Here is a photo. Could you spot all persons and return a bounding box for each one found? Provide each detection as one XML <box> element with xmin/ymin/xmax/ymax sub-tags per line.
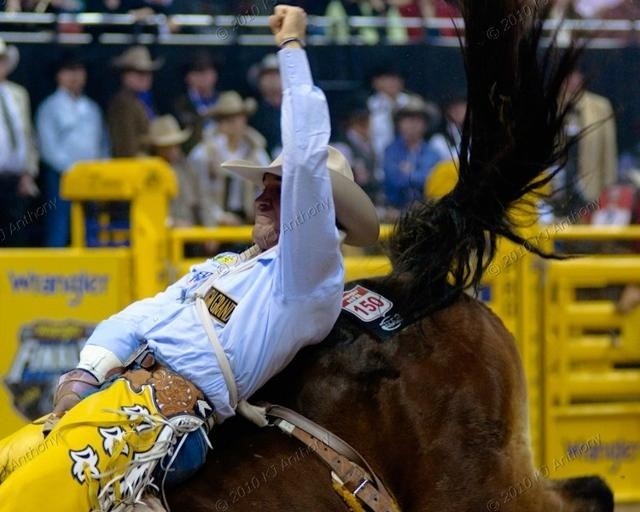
<box><xmin>0</xmin><ymin>0</ymin><xmax>639</xmax><ymax>314</ymax></box>
<box><xmin>0</xmin><ymin>4</ymin><xmax>380</xmax><ymax>512</ymax></box>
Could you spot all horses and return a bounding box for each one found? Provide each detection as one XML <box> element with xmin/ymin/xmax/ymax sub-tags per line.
<box><xmin>177</xmin><ymin>0</ymin><xmax>615</xmax><ymax>511</ymax></box>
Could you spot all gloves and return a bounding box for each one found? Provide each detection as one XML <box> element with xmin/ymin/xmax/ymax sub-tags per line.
<box><xmin>266</xmin><ymin>4</ymin><xmax>310</xmax><ymax>48</ymax></box>
<box><xmin>40</xmin><ymin>367</ymin><xmax>102</xmax><ymax>439</ymax></box>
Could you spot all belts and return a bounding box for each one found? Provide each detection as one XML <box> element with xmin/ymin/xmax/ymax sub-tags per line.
<box><xmin>121</xmin><ymin>340</ymin><xmax>159</xmax><ymax>377</ymax></box>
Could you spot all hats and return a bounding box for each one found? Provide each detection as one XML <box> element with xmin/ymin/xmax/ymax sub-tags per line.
<box><xmin>244</xmin><ymin>51</ymin><xmax>283</xmax><ymax>83</ymax></box>
<box><xmin>195</xmin><ymin>90</ymin><xmax>259</xmax><ymax>121</ymax></box>
<box><xmin>389</xmin><ymin>92</ymin><xmax>443</xmax><ymax>134</ymax></box>
<box><xmin>108</xmin><ymin>42</ymin><xmax>168</xmax><ymax>73</ymax></box>
<box><xmin>217</xmin><ymin>141</ymin><xmax>381</xmax><ymax>248</ymax></box>
<box><xmin>0</xmin><ymin>39</ymin><xmax>23</xmax><ymax>79</ymax></box>
<box><xmin>136</xmin><ymin>112</ymin><xmax>198</xmax><ymax>148</ymax></box>
